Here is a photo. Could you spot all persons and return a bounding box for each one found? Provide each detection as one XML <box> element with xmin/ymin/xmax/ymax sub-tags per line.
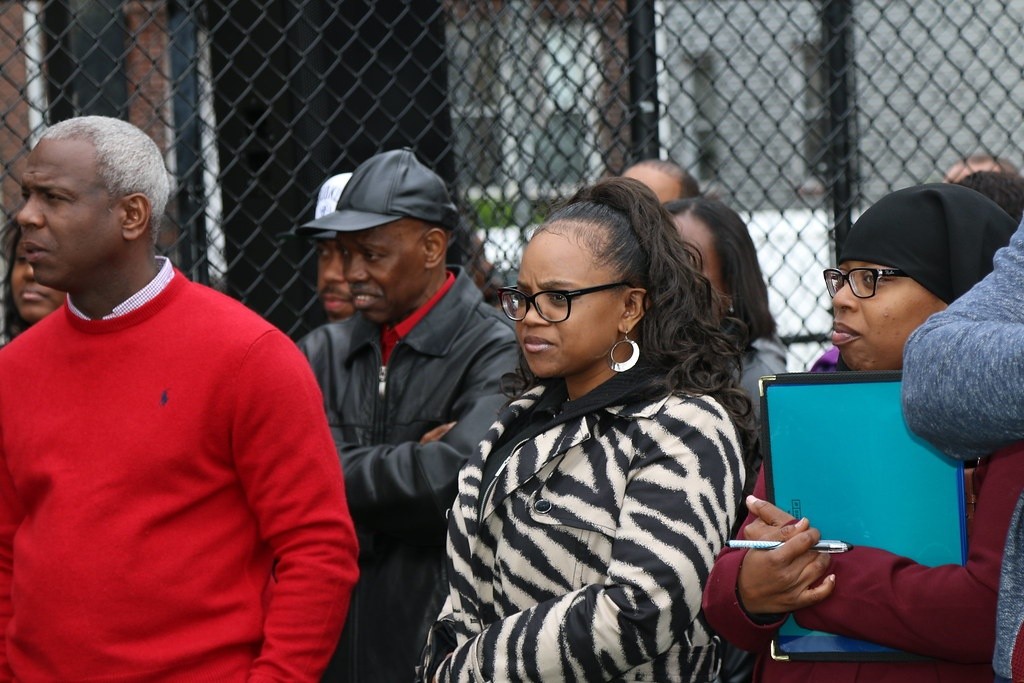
<box><xmin>619</xmin><ymin>160</ymin><xmax>787</xmax><ymax>461</ymax></box>
<box><xmin>702</xmin><ymin>182</ymin><xmax>1024</xmax><ymax>683</ymax></box>
<box><xmin>418</xmin><ymin>178</ymin><xmax>762</xmax><ymax>683</ymax></box>
<box><xmin>0</xmin><ymin>116</ymin><xmax>358</xmax><ymax>683</ymax></box>
<box><xmin>293</xmin><ymin>146</ymin><xmax>532</xmax><ymax>683</ymax></box>
<box><xmin>943</xmin><ymin>157</ymin><xmax>1024</xmax><ymax>223</ymax></box>
<box><xmin>900</xmin><ymin>206</ymin><xmax>1024</xmax><ymax>683</ymax></box>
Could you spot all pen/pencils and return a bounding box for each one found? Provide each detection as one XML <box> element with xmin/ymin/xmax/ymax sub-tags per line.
<box><xmin>723</xmin><ymin>538</ymin><xmax>853</xmax><ymax>554</ymax></box>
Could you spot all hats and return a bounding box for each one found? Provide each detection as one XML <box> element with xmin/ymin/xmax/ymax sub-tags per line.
<box><xmin>838</xmin><ymin>183</ymin><xmax>1020</xmax><ymax>307</ymax></box>
<box><xmin>277</xmin><ymin>171</ymin><xmax>354</xmax><ymax>241</ymax></box>
<box><xmin>296</xmin><ymin>146</ymin><xmax>461</xmax><ymax>231</ymax></box>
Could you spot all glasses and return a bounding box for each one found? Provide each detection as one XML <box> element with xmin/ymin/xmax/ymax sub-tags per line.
<box><xmin>497</xmin><ymin>282</ymin><xmax>648</xmax><ymax>323</ymax></box>
<box><xmin>824</xmin><ymin>268</ymin><xmax>910</xmax><ymax>299</ymax></box>
